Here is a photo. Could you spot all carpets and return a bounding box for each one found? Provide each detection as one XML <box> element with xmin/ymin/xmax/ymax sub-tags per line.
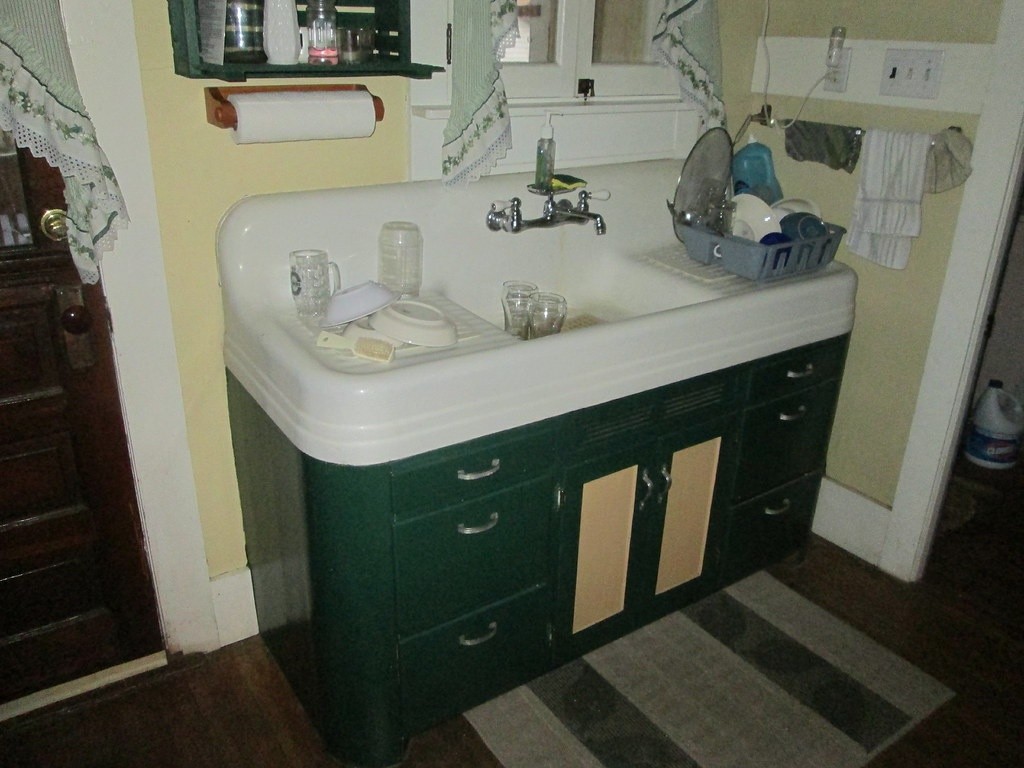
<box><xmin>461</xmin><ymin>569</ymin><xmax>962</xmax><ymax>767</ymax></box>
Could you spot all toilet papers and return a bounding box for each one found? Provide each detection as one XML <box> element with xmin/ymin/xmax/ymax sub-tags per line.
<box><xmin>227</xmin><ymin>89</ymin><xmax>378</xmax><ymax>141</ymax></box>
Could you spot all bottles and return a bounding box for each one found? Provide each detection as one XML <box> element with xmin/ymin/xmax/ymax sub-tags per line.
<box><xmin>305</xmin><ymin>0</ymin><xmax>339</xmax><ymax>56</ymax></box>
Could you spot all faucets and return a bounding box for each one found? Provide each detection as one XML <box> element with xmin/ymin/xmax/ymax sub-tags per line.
<box><xmin>542</xmin><ymin>190</ymin><xmax>606</xmax><ymax>234</ymax></box>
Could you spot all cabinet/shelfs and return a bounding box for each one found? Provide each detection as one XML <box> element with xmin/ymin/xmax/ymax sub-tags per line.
<box><xmin>218</xmin><ymin>336</ymin><xmax>853</xmax><ymax>768</ymax></box>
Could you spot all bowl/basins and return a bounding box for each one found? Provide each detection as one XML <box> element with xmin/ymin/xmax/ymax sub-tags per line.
<box><xmin>760</xmin><ymin>234</ymin><xmax>790</xmax><ymax>269</ymax></box>
<box><xmin>780</xmin><ymin>211</ymin><xmax>830</xmax><ymax>243</ymax></box>
<box><xmin>771</xmin><ymin>197</ymin><xmax>822</xmax><ymax>224</ymax></box>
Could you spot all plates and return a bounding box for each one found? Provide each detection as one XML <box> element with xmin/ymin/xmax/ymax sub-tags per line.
<box><xmin>373</xmin><ymin>294</ymin><xmax>459</xmax><ymax>348</ymax></box>
<box><xmin>730</xmin><ymin>194</ymin><xmax>782</xmax><ymax>242</ymax></box>
<box><xmin>321</xmin><ymin>280</ymin><xmax>403</xmax><ymax>328</ymax></box>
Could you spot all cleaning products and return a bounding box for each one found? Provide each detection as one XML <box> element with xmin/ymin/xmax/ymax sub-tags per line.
<box><xmin>963</xmin><ymin>379</ymin><xmax>1024</xmax><ymax>469</ymax></box>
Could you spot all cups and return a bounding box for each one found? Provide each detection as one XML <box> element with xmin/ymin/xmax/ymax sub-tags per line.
<box><xmin>336</xmin><ymin>27</ymin><xmax>376</xmax><ymax>65</ymax></box>
<box><xmin>502</xmin><ymin>277</ymin><xmax>539</xmax><ymax>341</ymax></box>
<box><xmin>529</xmin><ymin>292</ymin><xmax>568</xmax><ymax>338</ymax></box>
<box><xmin>286</xmin><ymin>248</ymin><xmax>341</xmax><ymax>318</ymax></box>
<box><xmin>380</xmin><ymin>221</ymin><xmax>424</xmax><ymax>300</ymax></box>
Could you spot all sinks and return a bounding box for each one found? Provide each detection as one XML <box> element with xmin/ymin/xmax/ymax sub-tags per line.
<box><xmin>427</xmin><ymin>237</ymin><xmax>716</xmax><ymax>348</ymax></box>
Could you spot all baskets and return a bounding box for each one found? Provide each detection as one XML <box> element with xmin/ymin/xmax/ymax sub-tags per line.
<box><xmin>677</xmin><ymin>219</ymin><xmax>847</xmax><ymax>282</ymax></box>
